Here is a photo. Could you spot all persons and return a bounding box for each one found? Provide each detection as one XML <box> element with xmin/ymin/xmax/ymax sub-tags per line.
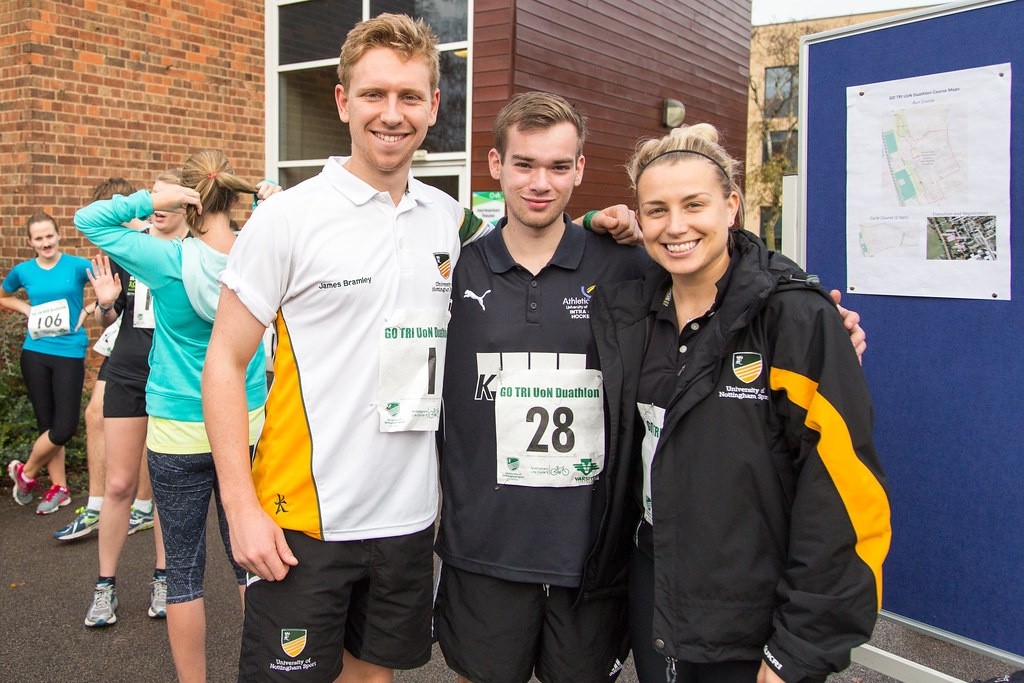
<box><xmin>201</xmin><ymin>12</ymin><xmax>644</xmax><ymax>683</ymax></box>
<box><xmin>570</xmin><ymin>123</ymin><xmax>893</xmax><ymax>683</ymax></box>
<box><xmin>74</xmin><ymin>151</ymin><xmax>282</xmax><ymax>682</ymax></box>
<box><xmin>435</xmin><ymin>91</ymin><xmax>866</xmax><ymax>683</ymax></box>
<box><xmin>0</xmin><ymin>169</ymin><xmax>195</xmax><ymax>626</ymax></box>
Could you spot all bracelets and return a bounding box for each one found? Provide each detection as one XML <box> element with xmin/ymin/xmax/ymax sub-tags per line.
<box><xmin>583</xmin><ymin>210</ymin><xmax>599</xmax><ymax>232</ymax></box>
<box><xmin>99</xmin><ymin>305</ymin><xmax>112</xmax><ymax>314</ymax></box>
<box><xmin>84</xmin><ymin>307</ymin><xmax>90</xmax><ymax>315</ymax></box>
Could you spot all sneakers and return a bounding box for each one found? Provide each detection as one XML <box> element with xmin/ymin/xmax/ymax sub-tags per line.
<box><xmin>53</xmin><ymin>507</ymin><xmax>100</xmax><ymax>541</ymax></box>
<box><xmin>36</xmin><ymin>484</ymin><xmax>72</xmax><ymax>515</ymax></box>
<box><xmin>84</xmin><ymin>578</ymin><xmax>118</xmax><ymax>627</ymax></box>
<box><xmin>127</xmin><ymin>504</ymin><xmax>154</xmax><ymax>535</ymax></box>
<box><xmin>148</xmin><ymin>570</ymin><xmax>166</xmax><ymax>618</ymax></box>
<box><xmin>8</xmin><ymin>460</ymin><xmax>38</xmax><ymax>506</ymax></box>
